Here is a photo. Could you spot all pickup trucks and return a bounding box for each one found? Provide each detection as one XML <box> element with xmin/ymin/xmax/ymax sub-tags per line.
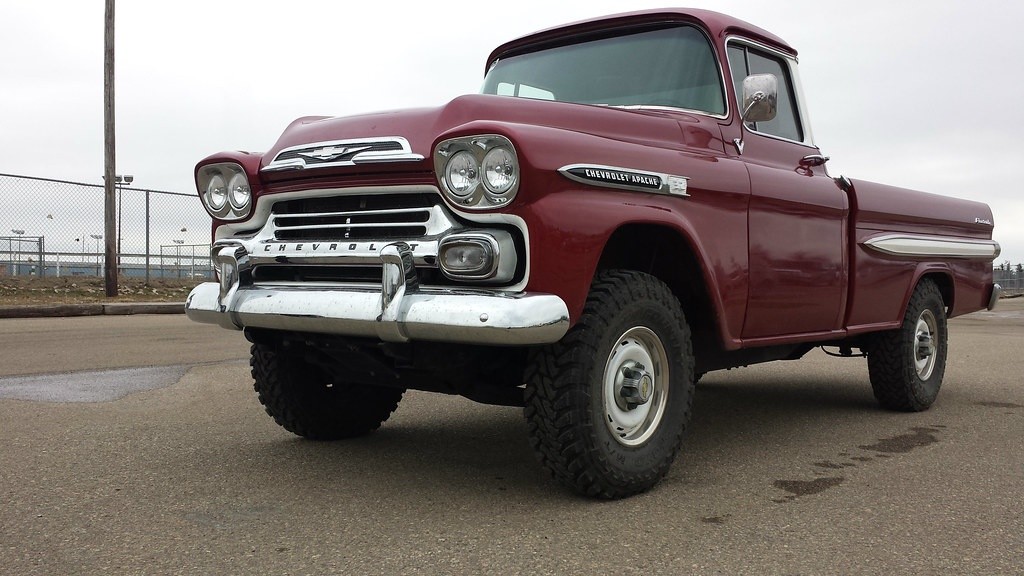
<box><xmin>183</xmin><ymin>8</ymin><xmax>1002</xmax><ymax>503</ymax></box>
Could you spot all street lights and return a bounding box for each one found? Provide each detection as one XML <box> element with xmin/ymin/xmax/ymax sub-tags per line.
<box><xmin>11</xmin><ymin>229</ymin><xmax>25</xmax><ymax>275</ymax></box>
<box><xmin>91</xmin><ymin>234</ymin><xmax>103</xmax><ymax>277</ymax></box>
<box><xmin>173</xmin><ymin>239</ymin><xmax>184</xmax><ymax>281</ymax></box>
<box><xmin>46</xmin><ymin>214</ymin><xmax>53</xmax><ymax>260</ymax></box>
<box><xmin>180</xmin><ymin>228</ymin><xmax>188</xmax><ymax>279</ymax></box>
<box><xmin>103</xmin><ymin>175</ymin><xmax>133</xmax><ymax>275</ymax></box>
